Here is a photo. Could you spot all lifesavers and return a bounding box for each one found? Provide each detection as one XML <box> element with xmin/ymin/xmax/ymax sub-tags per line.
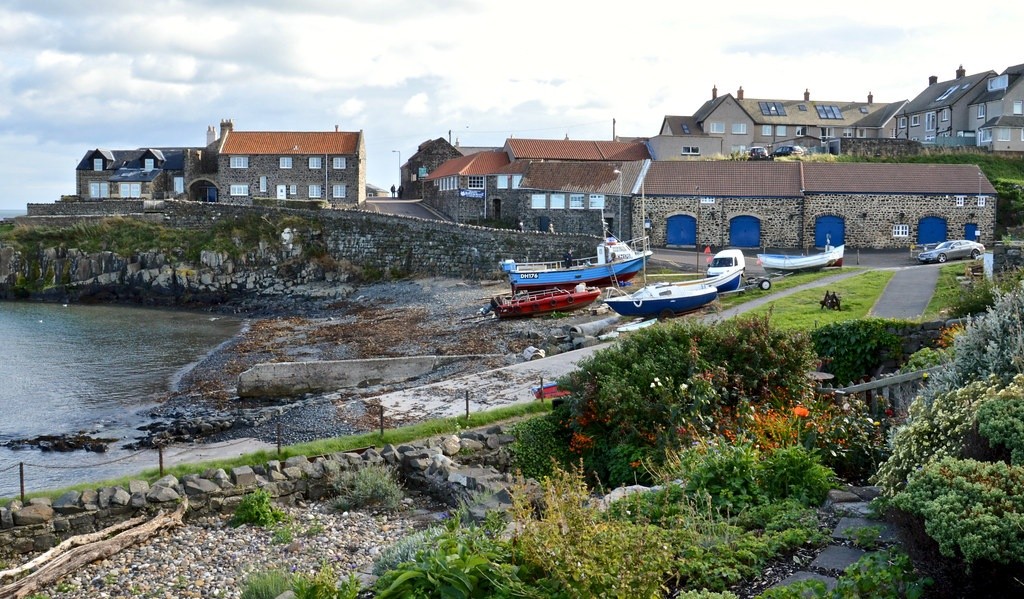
<box><xmin>515</xmin><ymin>306</ymin><xmax>522</xmax><ymax>313</ymax></box>
<box><xmin>532</xmin><ymin>302</ymin><xmax>540</xmax><ymax>310</ymax></box>
<box><xmin>549</xmin><ymin>299</ymin><xmax>557</xmax><ymax>307</ymax></box>
<box><xmin>481</xmin><ymin>303</ymin><xmax>493</xmax><ymax>315</ymax></box>
<box><xmin>566</xmin><ymin>296</ymin><xmax>575</xmax><ymax>303</ymax></box>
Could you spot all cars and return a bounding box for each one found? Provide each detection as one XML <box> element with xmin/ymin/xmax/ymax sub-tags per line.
<box><xmin>768</xmin><ymin>145</ymin><xmax>805</xmax><ymax>160</ymax></box>
<box><xmin>918</xmin><ymin>240</ymin><xmax>986</xmax><ymax>262</ymax></box>
<box><xmin>747</xmin><ymin>146</ymin><xmax>769</xmax><ymax>160</ymax></box>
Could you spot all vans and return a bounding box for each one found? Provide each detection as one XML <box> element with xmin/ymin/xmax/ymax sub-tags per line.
<box><xmin>707</xmin><ymin>249</ymin><xmax>746</xmax><ymax>279</ymax></box>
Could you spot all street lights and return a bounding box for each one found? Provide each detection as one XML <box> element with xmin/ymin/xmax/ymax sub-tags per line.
<box><xmin>613</xmin><ymin>170</ymin><xmax>622</xmax><ymax>243</ymax></box>
<box><xmin>392</xmin><ymin>150</ymin><xmax>400</xmax><ymax>187</ymax></box>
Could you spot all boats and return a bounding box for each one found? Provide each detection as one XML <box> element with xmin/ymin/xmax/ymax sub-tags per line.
<box><xmin>499</xmin><ymin>237</ymin><xmax>653</xmax><ymax>288</ymax></box>
<box><xmin>756</xmin><ymin>243</ymin><xmax>844</xmax><ymax>275</ymax></box>
<box><xmin>650</xmin><ymin>266</ymin><xmax>744</xmax><ymax>294</ymax></box>
<box><xmin>603</xmin><ymin>281</ymin><xmax>718</xmax><ymax>318</ymax></box>
<box><xmin>490</xmin><ymin>282</ymin><xmax>602</xmax><ymax>319</ymax></box>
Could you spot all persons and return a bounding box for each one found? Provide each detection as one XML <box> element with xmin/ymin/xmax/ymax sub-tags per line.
<box><xmin>390</xmin><ymin>184</ymin><xmax>396</xmax><ymax>198</ymax></box>
<box><xmin>397</xmin><ymin>185</ymin><xmax>404</xmax><ymax>199</ymax></box>
<box><xmin>564</xmin><ymin>248</ymin><xmax>573</xmax><ymax>269</ymax></box>
